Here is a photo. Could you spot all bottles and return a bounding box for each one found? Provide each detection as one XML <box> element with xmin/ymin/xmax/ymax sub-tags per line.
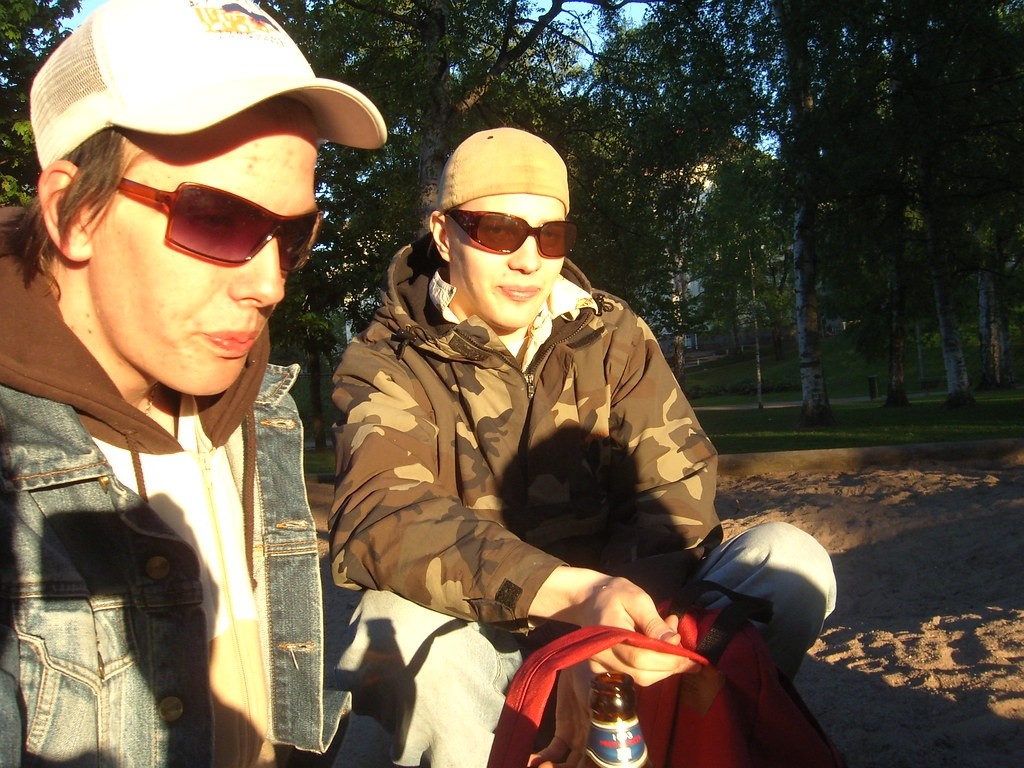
<box><xmin>576</xmin><ymin>670</ymin><xmax>653</xmax><ymax>768</ymax></box>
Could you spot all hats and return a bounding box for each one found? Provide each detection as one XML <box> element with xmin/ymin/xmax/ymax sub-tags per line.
<box><xmin>437</xmin><ymin>128</ymin><xmax>570</xmax><ymax>217</ymax></box>
<box><xmin>30</xmin><ymin>0</ymin><xmax>388</xmax><ymax>171</ymax></box>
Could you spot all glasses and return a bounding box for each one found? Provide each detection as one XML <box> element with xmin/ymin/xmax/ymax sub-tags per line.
<box><xmin>444</xmin><ymin>209</ymin><xmax>578</xmax><ymax>257</ymax></box>
<box><xmin>77</xmin><ymin>164</ymin><xmax>325</xmax><ymax>272</ymax></box>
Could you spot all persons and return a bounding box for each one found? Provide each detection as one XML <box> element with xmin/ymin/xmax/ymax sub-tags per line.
<box><xmin>0</xmin><ymin>0</ymin><xmax>388</xmax><ymax>768</ymax></box>
<box><xmin>328</xmin><ymin>126</ymin><xmax>837</xmax><ymax>767</ymax></box>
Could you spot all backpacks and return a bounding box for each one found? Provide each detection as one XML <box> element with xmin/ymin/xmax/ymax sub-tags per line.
<box><xmin>487</xmin><ymin>580</ymin><xmax>846</xmax><ymax>768</ymax></box>
